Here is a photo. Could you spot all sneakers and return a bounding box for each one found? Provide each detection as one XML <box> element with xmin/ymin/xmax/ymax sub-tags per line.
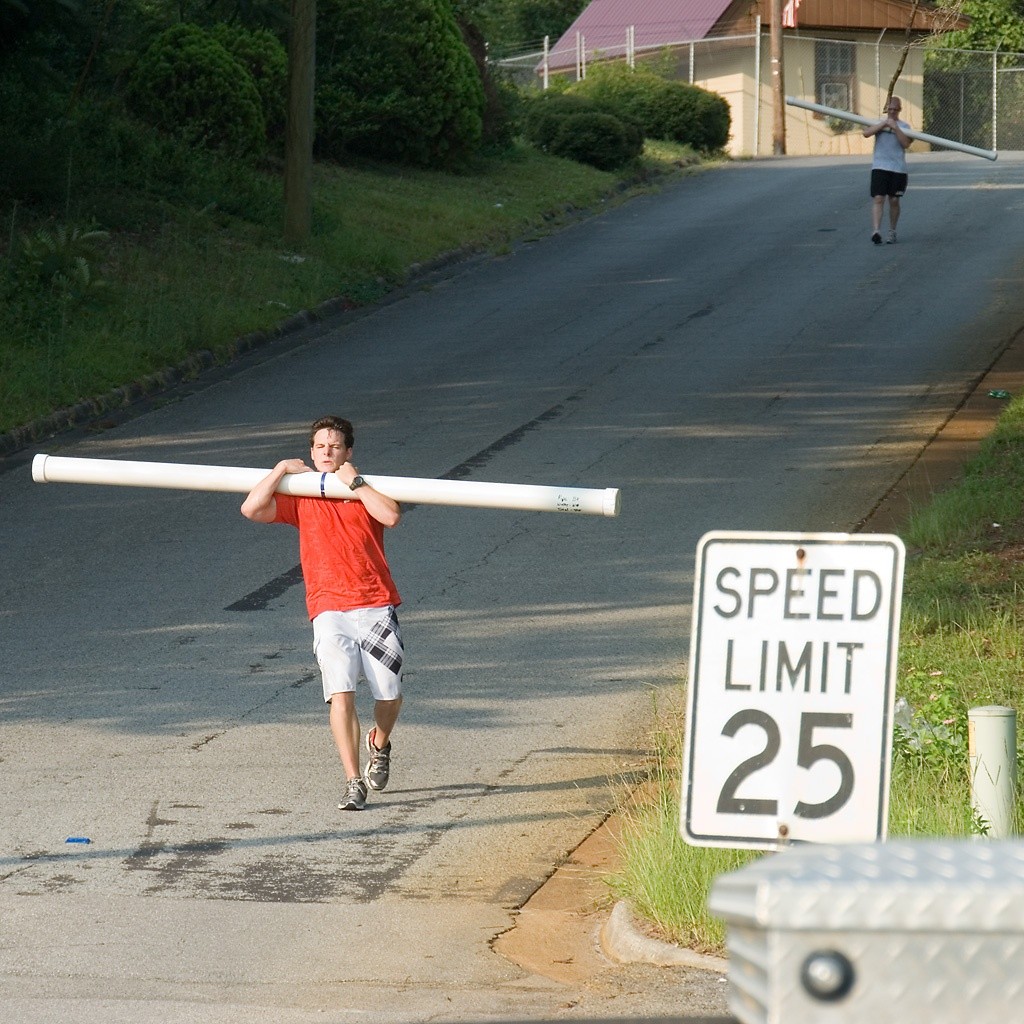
<box><xmin>872</xmin><ymin>232</ymin><xmax>883</xmax><ymax>244</ymax></box>
<box><xmin>886</xmin><ymin>229</ymin><xmax>895</xmax><ymax>243</ymax></box>
<box><xmin>364</xmin><ymin>725</ymin><xmax>391</xmax><ymax>791</ymax></box>
<box><xmin>337</xmin><ymin>778</ymin><xmax>369</xmax><ymax>811</ymax></box>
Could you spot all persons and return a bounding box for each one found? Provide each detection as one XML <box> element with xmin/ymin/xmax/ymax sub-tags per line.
<box><xmin>241</xmin><ymin>414</ymin><xmax>405</xmax><ymax>812</ymax></box>
<box><xmin>862</xmin><ymin>96</ymin><xmax>914</xmax><ymax>244</ymax></box>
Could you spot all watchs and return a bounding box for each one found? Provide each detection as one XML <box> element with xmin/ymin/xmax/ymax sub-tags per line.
<box><xmin>349</xmin><ymin>475</ymin><xmax>363</xmax><ymax>491</ymax></box>
